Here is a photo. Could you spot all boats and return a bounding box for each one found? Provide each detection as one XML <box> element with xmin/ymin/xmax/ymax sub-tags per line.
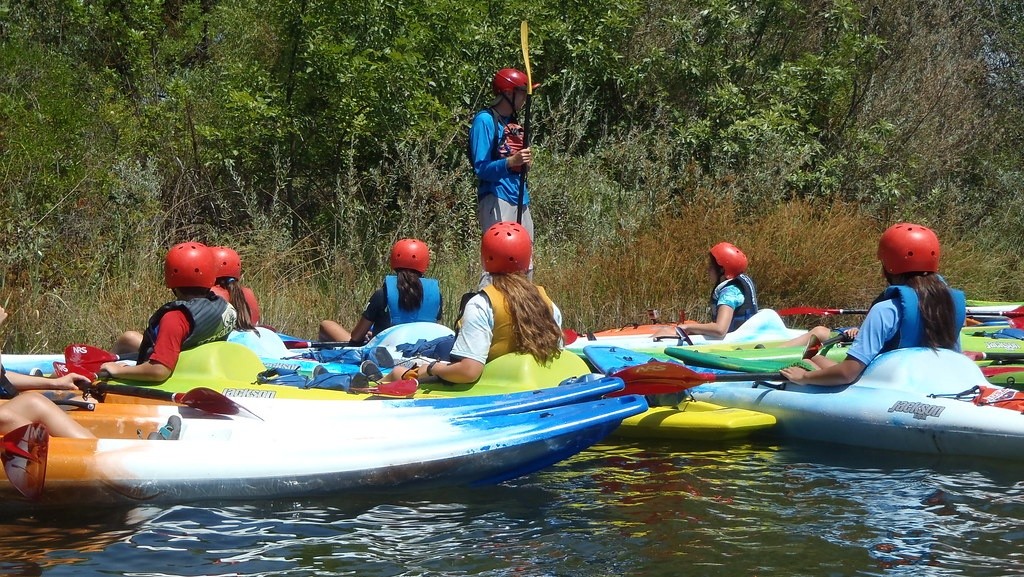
<box><xmin>0</xmin><ymin>299</ymin><xmax>1024</xmax><ymax>515</ymax></box>
<box><xmin>583</xmin><ymin>343</ymin><xmax>1024</xmax><ymax>459</ymax></box>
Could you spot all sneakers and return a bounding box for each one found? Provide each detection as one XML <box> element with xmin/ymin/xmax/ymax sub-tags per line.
<box><xmin>360</xmin><ymin>360</ymin><xmax>383</xmax><ymax>383</ymax></box>
<box><xmin>138</xmin><ymin>415</ymin><xmax>181</xmax><ymax>440</ymax></box>
<box><xmin>312</xmin><ymin>365</ymin><xmax>329</xmax><ymax>380</ymax></box>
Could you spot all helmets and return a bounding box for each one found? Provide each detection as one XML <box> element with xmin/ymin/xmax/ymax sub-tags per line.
<box><xmin>494</xmin><ymin>68</ymin><xmax>541</xmax><ymax>96</ymax></box>
<box><xmin>877</xmin><ymin>223</ymin><xmax>939</xmax><ymax>275</ymax></box>
<box><xmin>710</xmin><ymin>242</ymin><xmax>748</xmax><ymax>279</ymax></box>
<box><xmin>392</xmin><ymin>238</ymin><xmax>430</xmax><ymax>273</ymax></box>
<box><xmin>481</xmin><ymin>221</ymin><xmax>533</xmax><ymax>273</ymax></box>
<box><xmin>210</xmin><ymin>245</ymin><xmax>241</xmax><ymax>281</ymax></box>
<box><xmin>165</xmin><ymin>242</ymin><xmax>216</xmax><ymax>289</ymax></box>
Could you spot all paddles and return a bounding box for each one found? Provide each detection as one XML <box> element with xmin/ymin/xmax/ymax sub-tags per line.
<box><xmin>515</xmin><ymin>19</ymin><xmax>534</xmax><ymax>224</ymax></box>
<box><xmin>283</xmin><ymin>339</ymin><xmax>369</xmax><ymax>349</ymax></box>
<box><xmin>612</xmin><ymin>361</ymin><xmax>1023</xmax><ymax>394</ymax></box>
<box><xmin>801</xmin><ymin>333</ymin><xmax>847</xmax><ymax>360</ymax></box>
<box><xmin>74</xmin><ymin>379</ymin><xmax>266</xmax><ymax>423</ymax></box>
<box><xmin>777</xmin><ymin>306</ymin><xmax>1024</xmax><ymax>329</ymax></box>
<box><xmin>53</xmin><ymin>344</ymin><xmax>140</xmax><ymax>382</ymax></box>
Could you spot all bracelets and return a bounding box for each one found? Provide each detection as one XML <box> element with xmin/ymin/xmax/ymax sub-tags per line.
<box><xmin>428</xmin><ymin>361</ymin><xmax>439</xmax><ymax>376</ymax></box>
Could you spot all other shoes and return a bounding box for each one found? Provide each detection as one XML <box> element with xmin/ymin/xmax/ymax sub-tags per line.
<box><xmin>29</xmin><ymin>367</ymin><xmax>43</xmax><ymax>377</ymax></box>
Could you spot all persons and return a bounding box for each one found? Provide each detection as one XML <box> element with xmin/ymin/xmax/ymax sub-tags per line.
<box><xmin>414</xmin><ymin>222</ymin><xmax>563</xmax><ymax>384</ymax></box>
<box><xmin>782</xmin><ymin>221</ymin><xmax>967</xmax><ymax>388</ymax></box>
<box><xmin>206</xmin><ymin>242</ymin><xmax>261</xmax><ymax>329</ymax></box>
<box><xmin>101</xmin><ymin>242</ymin><xmax>237</xmax><ymax>381</ymax></box>
<box><xmin>470</xmin><ymin>68</ymin><xmax>534</xmax><ymax>284</ymax></box>
<box><xmin>679</xmin><ymin>240</ymin><xmax>757</xmax><ymax>339</ymax></box>
<box><xmin>0</xmin><ymin>307</ymin><xmax>183</xmax><ymax>439</ymax></box>
<box><xmin>317</xmin><ymin>237</ymin><xmax>441</xmax><ymax>347</ymax></box>
